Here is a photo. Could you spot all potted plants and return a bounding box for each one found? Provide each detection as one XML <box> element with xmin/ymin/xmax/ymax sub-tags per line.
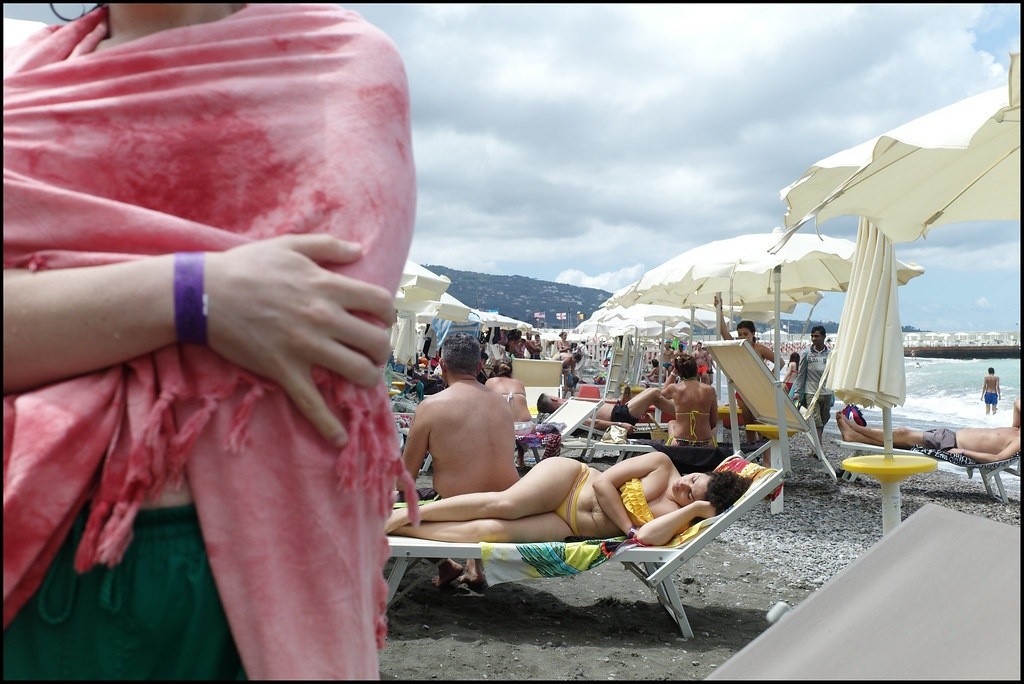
<box><xmin>593</xmin><ymin>372</ymin><xmax>608</xmax><ymax>385</ymax></box>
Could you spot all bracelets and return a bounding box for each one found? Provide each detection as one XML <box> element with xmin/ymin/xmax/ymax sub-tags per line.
<box><xmin>174</xmin><ymin>251</ymin><xmax>207</xmax><ymax>345</ymax></box>
<box><xmin>627</xmin><ymin>525</ymin><xmax>637</xmax><ymax>539</ymax></box>
<box><xmin>960</xmin><ymin>449</ymin><xmax>966</xmax><ymax>455</ymax></box>
<box><xmin>619</xmin><ymin>422</ymin><xmax>621</xmax><ymax>426</ymax></box>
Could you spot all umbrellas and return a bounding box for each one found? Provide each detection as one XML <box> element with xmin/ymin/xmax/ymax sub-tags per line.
<box><xmin>393</xmin><ymin>259</ymin><xmax>533</xmax><ymax>378</ymax></box>
<box><xmin>629</xmin><ymin>225</ymin><xmax>925</xmax><ymax>381</ymax></box>
<box><xmin>801</xmin><ymin>221</ymin><xmax>906</xmax><ymax>459</ymax></box>
<box><xmin>574</xmin><ymin>280</ymin><xmax>736</xmax><ymax>423</ymax></box>
<box><xmin>767</xmin><ymin>52</ymin><xmax>1020</xmax><ymax>254</ymax></box>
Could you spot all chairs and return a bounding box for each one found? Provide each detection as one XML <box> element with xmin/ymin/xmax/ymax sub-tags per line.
<box><xmin>387</xmin><ymin>358</ymin><xmax>1020</xmax><ymax>680</ymax></box>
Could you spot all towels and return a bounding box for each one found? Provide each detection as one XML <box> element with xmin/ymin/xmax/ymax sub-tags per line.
<box><xmin>649</xmin><ymin>438</ymin><xmax>769</xmax><ymax>473</ymax></box>
<box><xmin>913</xmin><ymin>444</ymin><xmax>1020</xmax><ymax>478</ymax></box>
<box><xmin>479</xmin><ymin>459</ymin><xmax>783</xmax><ymax>587</ymax></box>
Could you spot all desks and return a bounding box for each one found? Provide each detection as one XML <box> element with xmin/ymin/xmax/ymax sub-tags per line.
<box><xmin>718</xmin><ymin>407</ymin><xmax>744</xmax><ymax>443</ymax></box>
<box><xmin>842</xmin><ymin>455</ymin><xmax>938</xmax><ymax>537</ymax></box>
<box><xmin>745</xmin><ymin>424</ymin><xmax>800</xmax><ymax>514</ymax></box>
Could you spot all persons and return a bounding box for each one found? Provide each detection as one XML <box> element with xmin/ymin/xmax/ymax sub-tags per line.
<box><xmin>383</xmin><ymin>451</ymin><xmax>753</xmax><ymax>546</ymax></box>
<box><xmin>714</xmin><ymin>295</ymin><xmax>785</xmax><ymax>445</ymax></box>
<box><xmin>836</xmin><ymin>397</ymin><xmax>1020</xmax><ymax>464</ymax></box>
<box><xmin>401</xmin><ymin>334</ymin><xmax>520</xmax><ymax>498</ymax></box>
<box><xmin>981</xmin><ymin>368</ymin><xmax>1001</xmax><ymax>415</ymax></box>
<box><xmin>4</xmin><ymin>4</ymin><xmax>417</xmax><ymax>680</ymax></box>
<box><xmin>403</xmin><ymin>325</ymin><xmax>833</xmax><ymax>459</ymax></box>
